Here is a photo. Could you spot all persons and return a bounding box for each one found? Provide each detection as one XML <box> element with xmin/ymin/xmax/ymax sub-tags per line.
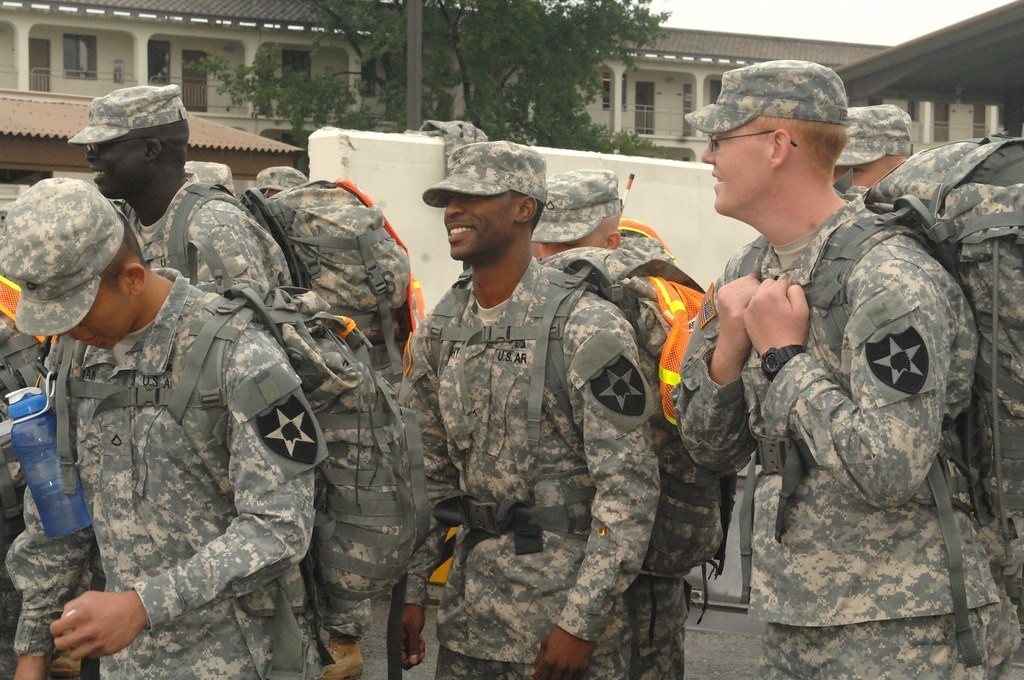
<box><xmin>833</xmin><ymin>104</ymin><xmax>913</xmax><ymax>189</ymax></box>
<box><xmin>256</xmin><ymin>166</ymin><xmax>372</xmax><ymax>680</ymax></box>
<box><xmin>183</xmin><ymin>162</ymin><xmax>235</xmax><ymax>197</ymax></box>
<box><xmin>0</xmin><ymin>177</ymin><xmax>330</xmax><ymax>680</ymax></box>
<box><xmin>66</xmin><ymin>84</ymin><xmax>293</xmax><ymax>304</ymax></box>
<box><xmin>529</xmin><ymin>170</ymin><xmax>690</xmax><ymax>680</ymax></box>
<box><xmin>399</xmin><ymin>139</ymin><xmax>661</xmax><ymax>680</ymax></box>
<box><xmin>667</xmin><ymin>59</ymin><xmax>1022</xmax><ymax>680</ymax></box>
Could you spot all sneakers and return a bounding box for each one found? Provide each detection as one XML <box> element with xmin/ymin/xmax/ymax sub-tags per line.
<box><xmin>321</xmin><ymin>633</ymin><xmax>364</xmax><ymax>680</ymax></box>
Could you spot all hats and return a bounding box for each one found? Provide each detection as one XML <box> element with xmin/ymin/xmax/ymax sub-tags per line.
<box><xmin>0</xmin><ymin>178</ymin><xmax>124</xmax><ymax>337</ymax></box>
<box><xmin>422</xmin><ymin>141</ymin><xmax>548</xmax><ymax>207</ymax></box>
<box><xmin>836</xmin><ymin>105</ymin><xmax>913</xmax><ymax>166</ymax></box>
<box><xmin>256</xmin><ymin>166</ymin><xmax>308</xmax><ymax>190</ymax></box>
<box><xmin>68</xmin><ymin>83</ymin><xmax>188</xmax><ymax>146</ymax></box>
<box><xmin>685</xmin><ymin>60</ymin><xmax>848</xmax><ymax>136</ymax></box>
<box><xmin>532</xmin><ymin>170</ymin><xmax>621</xmax><ymax>243</ymax></box>
<box><xmin>185</xmin><ymin>161</ymin><xmax>236</xmax><ymax>197</ymax></box>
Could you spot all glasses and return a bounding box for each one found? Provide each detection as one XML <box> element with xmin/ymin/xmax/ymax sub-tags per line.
<box><xmin>87</xmin><ymin>135</ymin><xmax>166</xmax><ymax>155</ymax></box>
<box><xmin>709</xmin><ymin>130</ymin><xmax>797</xmax><ymax>152</ymax></box>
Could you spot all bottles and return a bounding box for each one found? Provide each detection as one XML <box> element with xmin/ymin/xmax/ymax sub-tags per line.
<box><xmin>4</xmin><ymin>371</ymin><xmax>91</xmax><ymax>537</ymax></box>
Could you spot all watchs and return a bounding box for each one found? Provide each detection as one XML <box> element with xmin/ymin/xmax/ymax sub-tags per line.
<box><xmin>761</xmin><ymin>343</ymin><xmax>805</xmax><ymax>382</ymax></box>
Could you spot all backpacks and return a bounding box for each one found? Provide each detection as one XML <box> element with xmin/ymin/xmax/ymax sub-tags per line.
<box><xmin>58</xmin><ymin>280</ymin><xmax>418</xmax><ymax>598</ymax></box>
<box><xmin>425</xmin><ymin>247</ymin><xmax>723</xmax><ymax>578</ymax></box>
<box><xmin>739</xmin><ymin>138</ymin><xmax>1024</xmax><ymax>665</ymax></box>
<box><xmin>172</xmin><ymin>181</ymin><xmax>412</xmax><ymax>375</ymax></box>
